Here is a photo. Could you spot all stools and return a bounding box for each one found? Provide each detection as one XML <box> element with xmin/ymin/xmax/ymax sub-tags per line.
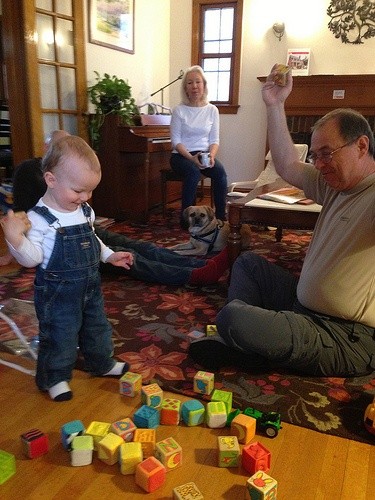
<box><xmin>159</xmin><ymin>169</ymin><xmax>214</xmax><ymax>218</ymax></box>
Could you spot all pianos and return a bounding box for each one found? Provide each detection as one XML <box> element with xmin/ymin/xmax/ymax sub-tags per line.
<box><xmin>89</xmin><ymin>113</ymin><xmax>181</xmax><ymax>224</ymax></box>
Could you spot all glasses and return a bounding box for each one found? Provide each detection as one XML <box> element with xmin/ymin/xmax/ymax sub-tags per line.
<box><xmin>306</xmin><ymin>137</ymin><xmax>361</xmax><ymax>163</ymax></box>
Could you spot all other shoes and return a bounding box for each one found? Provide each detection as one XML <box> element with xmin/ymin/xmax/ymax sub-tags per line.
<box><xmin>180</xmin><ymin>214</ymin><xmax>187</xmax><ymax>227</ymax></box>
<box><xmin>188</xmin><ymin>335</ymin><xmax>273</xmax><ymax>375</ymax></box>
<box><xmin>216</xmin><ymin>217</ymin><xmax>227</xmax><ymax>221</ymax></box>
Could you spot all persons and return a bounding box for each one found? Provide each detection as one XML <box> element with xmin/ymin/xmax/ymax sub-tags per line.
<box><xmin>13</xmin><ymin>129</ymin><xmax>231</xmax><ymax>289</ymax></box>
<box><xmin>168</xmin><ymin>65</ymin><xmax>228</xmax><ymax>221</ymax></box>
<box><xmin>187</xmin><ymin>63</ymin><xmax>375</xmax><ymax>383</ymax></box>
<box><xmin>1</xmin><ymin>136</ymin><xmax>134</xmax><ymax>403</ymax></box>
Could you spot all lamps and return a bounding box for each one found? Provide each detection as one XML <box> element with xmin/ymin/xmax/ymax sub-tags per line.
<box><xmin>271</xmin><ymin>22</ymin><xmax>285</xmax><ymax>41</ymax></box>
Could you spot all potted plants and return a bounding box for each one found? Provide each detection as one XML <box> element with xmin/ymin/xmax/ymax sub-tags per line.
<box><xmin>85</xmin><ymin>70</ymin><xmax>140</xmax><ymax>152</ymax></box>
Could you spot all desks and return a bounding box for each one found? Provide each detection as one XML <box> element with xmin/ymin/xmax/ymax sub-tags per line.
<box><xmin>227</xmin><ymin>184</ymin><xmax>324</xmax><ymax>293</ymax></box>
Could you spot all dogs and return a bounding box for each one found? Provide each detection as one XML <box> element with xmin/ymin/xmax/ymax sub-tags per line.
<box><xmin>165</xmin><ymin>203</ymin><xmax>253</xmax><ymax>255</ymax></box>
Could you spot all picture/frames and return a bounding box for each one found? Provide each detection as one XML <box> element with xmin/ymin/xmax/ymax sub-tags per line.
<box><xmin>88</xmin><ymin>0</ymin><xmax>136</xmax><ymax>55</ymax></box>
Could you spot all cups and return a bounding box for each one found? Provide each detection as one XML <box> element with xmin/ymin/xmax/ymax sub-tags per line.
<box><xmin>200</xmin><ymin>153</ymin><xmax>210</xmax><ymax>167</ymax></box>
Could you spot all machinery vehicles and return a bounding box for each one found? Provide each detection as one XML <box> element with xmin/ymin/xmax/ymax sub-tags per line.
<box><xmin>226</xmin><ymin>406</ymin><xmax>283</xmax><ymax>439</ymax></box>
